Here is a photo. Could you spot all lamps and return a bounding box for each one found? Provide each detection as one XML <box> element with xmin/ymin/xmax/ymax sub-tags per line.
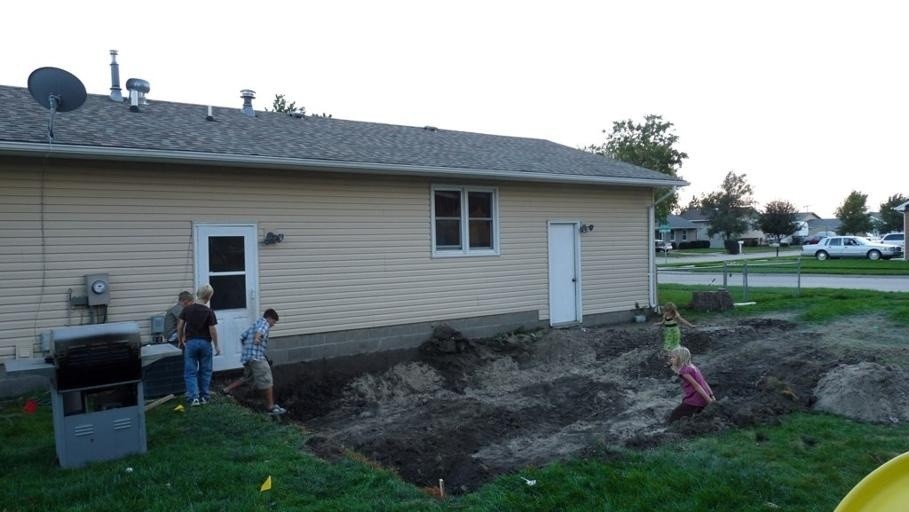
<box><xmin>264</xmin><ymin>232</ymin><xmax>284</xmax><ymax>246</ymax></box>
<box><xmin>580</xmin><ymin>224</ymin><xmax>594</xmax><ymax>234</ymax></box>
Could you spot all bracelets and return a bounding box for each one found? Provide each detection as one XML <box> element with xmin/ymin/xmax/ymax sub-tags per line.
<box><xmin>710</xmin><ymin>393</ymin><xmax>715</xmax><ymax>397</ymax></box>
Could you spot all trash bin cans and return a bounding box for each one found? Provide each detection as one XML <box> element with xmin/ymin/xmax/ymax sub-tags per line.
<box><xmin>729</xmin><ymin>243</ymin><xmax>739</xmax><ymax>254</ymax></box>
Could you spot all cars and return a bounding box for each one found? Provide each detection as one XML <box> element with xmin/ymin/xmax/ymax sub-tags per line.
<box><xmin>800</xmin><ymin>231</ymin><xmax>904</xmax><ymax>260</ymax></box>
<box><xmin>655</xmin><ymin>239</ymin><xmax>673</xmax><ymax>253</ymax></box>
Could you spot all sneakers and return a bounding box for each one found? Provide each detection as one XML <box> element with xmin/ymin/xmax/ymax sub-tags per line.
<box><xmin>221</xmin><ymin>389</ymin><xmax>236</xmax><ymax>399</ymax></box>
<box><xmin>192</xmin><ymin>397</ymin><xmax>209</xmax><ymax>406</ymax></box>
<box><xmin>264</xmin><ymin>405</ymin><xmax>286</xmax><ymax>415</ymax></box>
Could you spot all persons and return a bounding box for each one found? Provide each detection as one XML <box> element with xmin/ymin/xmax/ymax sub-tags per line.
<box><xmin>217</xmin><ymin>309</ymin><xmax>288</xmax><ymax>416</ymax></box>
<box><xmin>651</xmin><ymin>301</ymin><xmax>698</xmax><ymax>351</ymax></box>
<box><xmin>667</xmin><ymin>346</ymin><xmax>717</xmax><ymax>427</ymax></box>
<box><xmin>176</xmin><ymin>282</ymin><xmax>222</xmax><ymax>408</ymax></box>
<box><xmin>162</xmin><ymin>289</ymin><xmax>194</xmax><ymax>350</ymax></box>
